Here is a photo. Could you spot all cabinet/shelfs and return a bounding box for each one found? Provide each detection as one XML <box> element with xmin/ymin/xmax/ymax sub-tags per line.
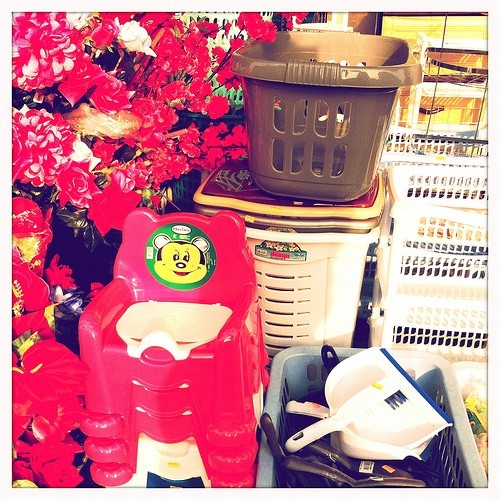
<box><xmin>367</xmin><ymin>156</ymin><xmax>488</xmax><ymax>363</ymax></box>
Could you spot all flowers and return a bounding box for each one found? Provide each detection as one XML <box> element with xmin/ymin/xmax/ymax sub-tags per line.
<box><xmin>13</xmin><ymin>12</ymin><xmax>310</xmax><ymax>350</ymax></box>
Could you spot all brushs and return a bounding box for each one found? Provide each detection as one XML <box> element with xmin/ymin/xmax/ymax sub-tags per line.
<box><xmin>284</xmin><ymin>377</ymin><xmax>408</xmax><ymax>458</ymax></box>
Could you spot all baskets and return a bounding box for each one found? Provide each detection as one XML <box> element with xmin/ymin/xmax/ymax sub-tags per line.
<box><xmin>383</xmin><ymin>126</ymin><xmax>488</xmax><ymax>166</ymax></box>
<box><xmin>229</xmin><ymin>30</ymin><xmax>413</xmax><ymax>202</ymax></box>
<box><xmin>196</xmin><ymin>205</ymin><xmax>381</xmax><ymax>367</ymax></box>
<box><xmin>172</xmin><ymin>11</ymin><xmax>271</xmax><ymax>116</ymax></box>
<box><xmin>255</xmin><ymin>345</ymin><xmax>489</xmax><ymax>488</ymax></box>
<box><xmin>376</xmin><ymin>164</ymin><xmax>487</xmax><ymax>293</ymax></box>
<box><xmin>368</xmin><ymin>272</ymin><xmax>488</xmax><ymax>349</ymax></box>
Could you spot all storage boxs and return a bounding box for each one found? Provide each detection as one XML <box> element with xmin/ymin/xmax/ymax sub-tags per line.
<box><xmin>249</xmin><ymin>345</ymin><xmax>489</xmax><ymax>488</ymax></box>
<box><xmin>191</xmin><ymin>158</ymin><xmax>386</xmax><ymax>373</ymax></box>
<box><xmin>227</xmin><ymin>29</ymin><xmax>423</xmax><ymax>203</ymax></box>
